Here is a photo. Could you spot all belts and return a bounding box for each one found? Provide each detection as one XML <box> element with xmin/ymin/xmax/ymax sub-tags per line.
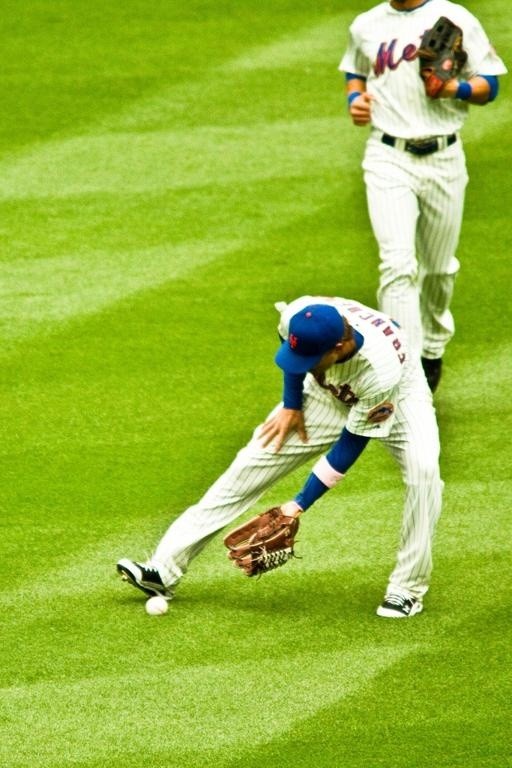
<box><xmin>382</xmin><ymin>133</ymin><xmax>455</xmax><ymax>155</ymax></box>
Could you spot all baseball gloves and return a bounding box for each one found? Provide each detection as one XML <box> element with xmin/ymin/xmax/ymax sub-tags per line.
<box><xmin>418</xmin><ymin>16</ymin><xmax>466</xmax><ymax>99</ymax></box>
<box><xmin>224</xmin><ymin>506</ymin><xmax>297</xmax><ymax>576</ymax></box>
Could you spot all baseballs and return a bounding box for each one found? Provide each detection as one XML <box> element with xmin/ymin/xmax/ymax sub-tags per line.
<box><xmin>146</xmin><ymin>596</ymin><xmax>167</xmax><ymax>615</ymax></box>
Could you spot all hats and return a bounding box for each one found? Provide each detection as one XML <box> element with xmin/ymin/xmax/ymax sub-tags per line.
<box><xmin>274</xmin><ymin>304</ymin><xmax>345</xmax><ymax>374</ymax></box>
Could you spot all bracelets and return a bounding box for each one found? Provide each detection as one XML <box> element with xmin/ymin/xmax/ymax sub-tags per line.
<box><xmin>345</xmin><ymin>92</ymin><xmax>360</xmax><ymax>104</ymax></box>
<box><xmin>454</xmin><ymin>82</ymin><xmax>471</xmax><ymax>102</ymax></box>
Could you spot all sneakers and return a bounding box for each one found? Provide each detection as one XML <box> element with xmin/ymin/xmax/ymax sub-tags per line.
<box><xmin>118</xmin><ymin>558</ymin><xmax>165</xmax><ymax>596</ymax></box>
<box><xmin>376</xmin><ymin>593</ymin><xmax>422</xmax><ymax>618</ymax></box>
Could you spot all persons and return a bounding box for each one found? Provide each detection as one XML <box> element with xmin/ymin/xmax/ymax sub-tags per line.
<box><xmin>335</xmin><ymin>0</ymin><xmax>509</xmax><ymax>398</ymax></box>
<box><xmin>115</xmin><ymin>293</ymin><xmax>448</xmax><ymax>620</ymax></box>
